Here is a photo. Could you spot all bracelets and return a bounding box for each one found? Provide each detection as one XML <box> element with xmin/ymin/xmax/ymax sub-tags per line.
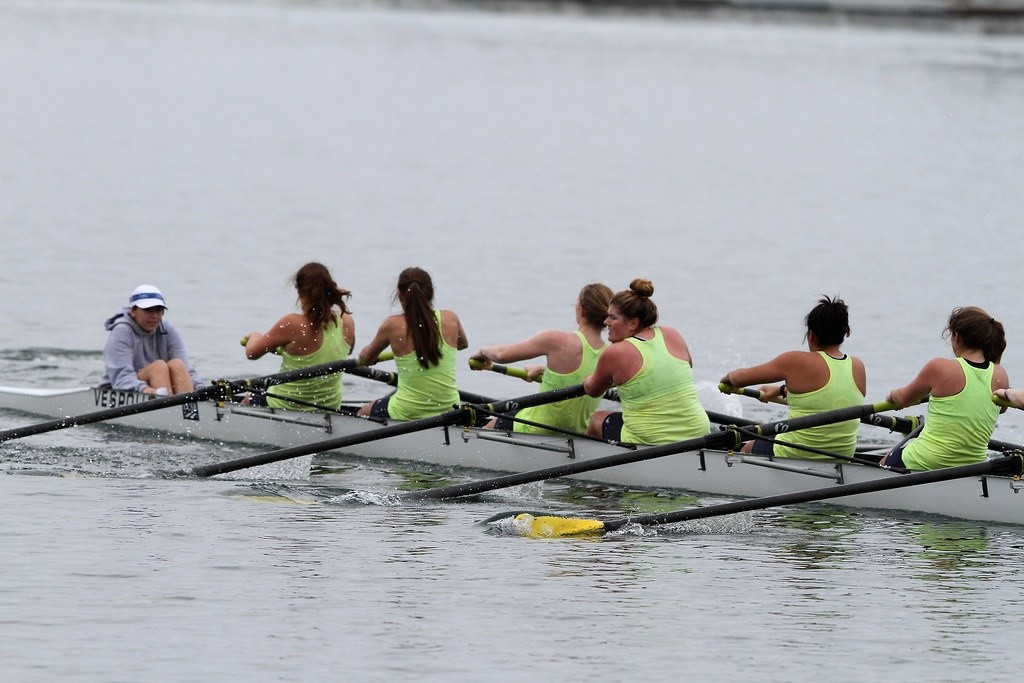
<box><xmin>1005</xmin><ymin>391</ymin><xmax>1009</xmax><ymax>401</ymax></box>
<box><xmin>779</xmin><ymin>385</ymin><xmax>787</xmax><ymax>398</ymax></box>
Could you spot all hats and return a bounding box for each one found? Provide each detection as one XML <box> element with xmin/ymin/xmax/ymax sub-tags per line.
<box><xmin>130</xmin><ymin>285</ymin><xmax>168</xmax><ymax>310</ymax></box>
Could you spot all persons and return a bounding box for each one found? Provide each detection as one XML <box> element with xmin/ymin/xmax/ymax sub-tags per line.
<box><xmin>990</xmin><ymin>388</ymin><xmax>1024</xmax><ymax>411</ymax></box>
<box><xmin>880</xmin><ymin>306</ymin><xmax>1010</xmax><ymax>474</ymax></box>
<box><xmin>719</xmin><ymin>293</ymin><xmax>866</xmax><ymax>463</ymax></box>
<box><xmin>242</xmin><ymin>262</ymin><xmax>355</xmax><ymax>413</ymax></box>
<box><xmin>99</xmin><ymin>284</ymin><xmax>205</xmax><ymax>401</ymax></box>
<box><xmin>355</xmin><ymin>267</ymin><xmax>469</xmax><ymax>421</ymax></box>
<box><xmin>582</xmin><ymin>277</ymin><xmax>710</xmax><ymax>447</ymax></box>
<box><xmin>468</xmin><ymin>283</ymin><xmax>613</xmax><ymax>435</ymax></box>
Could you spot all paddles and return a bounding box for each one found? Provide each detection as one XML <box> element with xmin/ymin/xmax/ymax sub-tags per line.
<box><xmin>0</xmin><ymin>349</ymin><xmax>396</xmax><ymax>444</ymax></box>
<box><xmin>468</xmin><ymin>356</ymin><xmax>767</xmax><ymax>428</ymax></box>
<box><xmin>240</xmin><ymin>336</ymin><xmax>502</xmax><ymax>406</ymax></box>
<box><xmin>412</xmin><ymin>395</ymin><xmax>930</xmax><ymax>499</ymax></box>
<box><xmin>717</xmin><ymin>382</ymin><xmax>1024</xmax><ymax>457</ymax></box>
<box><xmin>191</xmin><ymin>383</ymin><xmax>588</xmax><ymax>480</ymax></box>
<box><xmin>523</xmin><ymin>457</ymin><xmax>1024</xmax><ymax>540</ymax></box>
<box><xmin>991</xmin><ymin>393</ymin><xmax>1019</xmax><ymax>409</ymax></box>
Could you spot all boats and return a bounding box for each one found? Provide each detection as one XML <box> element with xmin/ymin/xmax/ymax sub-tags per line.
<box><xmin>0</xmin><ymin>383</ymin><xmax>1023</xmax><ymax>526</ymax></box>
